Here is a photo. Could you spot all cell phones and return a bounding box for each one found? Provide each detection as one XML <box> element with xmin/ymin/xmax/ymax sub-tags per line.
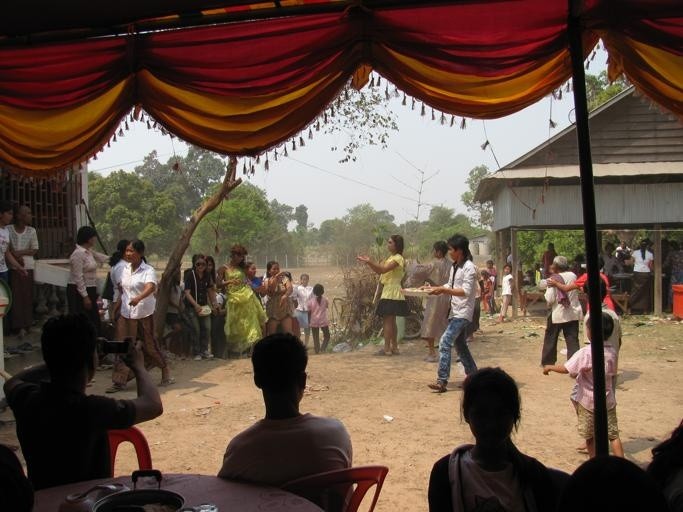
<box><xmin>103</xmin><ymin>341</ymin><xmax>128</xmax><ymax>353</ymax></box>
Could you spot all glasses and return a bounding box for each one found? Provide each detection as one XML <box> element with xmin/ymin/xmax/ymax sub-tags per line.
<box><xmin>194</xmin><ymin>263</ymin><xmax>206</xmax><ymax>266</ymax></box>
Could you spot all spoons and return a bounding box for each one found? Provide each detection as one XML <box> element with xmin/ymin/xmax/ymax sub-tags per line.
<box><xmin>66</xmin><ymin>482</ymin><xmax>123</xmax><ymax>502</ymax></box>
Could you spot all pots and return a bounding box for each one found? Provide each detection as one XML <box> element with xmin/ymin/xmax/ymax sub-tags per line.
<box><xmin>91</xmin><ymin>469</ymin><xmax>187</xmax><ymax>512</ymax></box>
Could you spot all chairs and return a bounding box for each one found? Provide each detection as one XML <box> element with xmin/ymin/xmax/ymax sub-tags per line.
<box><xmin>108</xmin><ymin>427</ymin><xmax>152</xmax><ymax>479</ymax></box>
<box><xmin>284</xmin><ymin>466</ymin><xmax>391</xmax><ymax>512</ymax></box>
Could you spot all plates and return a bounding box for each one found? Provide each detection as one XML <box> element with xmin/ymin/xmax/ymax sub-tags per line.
<box><xmin>400</xmin><ymin>287</ymin><xmax>436</xmax><ymax>296</ymax></box>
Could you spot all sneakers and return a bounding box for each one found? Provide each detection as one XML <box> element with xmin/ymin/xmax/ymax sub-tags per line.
<box><xmin>194</xmin><ymin>355</ymin><xmax>202</xmax><ymax>360</ymax></box>
<box><xmin>422</xmin><ymin>353</ymin><xmax>429</xmax><ymax>359</ymax></box>
<box><xmin>201</xmin><ymin>352</ymin><xmax>214</xmax><ymax>358</ymax></box>
<box><xmin>426</xmin><ymin>357</ymin><xmax>437</xmax><ymax>362</ymax></box>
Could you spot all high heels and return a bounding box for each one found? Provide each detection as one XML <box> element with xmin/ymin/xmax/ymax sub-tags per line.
<box><xmin>220</xmin><ymin>351</ymin><xmax>226</xmax><ymax>359</ymax></box>
<box><xmin>248</xmin><ymin>348</ymin><xmax>253</xmax><ymax>357</ymax></box>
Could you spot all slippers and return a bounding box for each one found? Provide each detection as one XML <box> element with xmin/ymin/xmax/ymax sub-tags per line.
<box><xmin>376</xmin><ymin>348</ymin><xmax>392</xmax><ymax>356</ymax></box>
<box><xmin>105</xmin><ymin>383</ymin><xmax>124</xmax><ymax>392</ymax></box>
<box><xmin>159</xmin><ymin>378</ymin><xmax>175</xmax><ymax>385</ymax></box>
<box><xmin>392</xmin><ymin>350</ymin><xmax>400</xmax><ymax>355</ymax></box>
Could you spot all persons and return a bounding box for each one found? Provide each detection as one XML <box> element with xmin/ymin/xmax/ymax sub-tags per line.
<box><xmin>5</xmin><ymin>315</ymin><xmax>161</xmax><ymax>490</ymax></box>
<box><xmin>498</xmin><ymin>265</ymin><xmax>514</xmax><ymax>322</ymax></box>
<box><xmin>184</xmin><ymin>253</ymin><xmax>213</xmax><ymax>361</ymax></box>
<box><xmin>358</xmin><ymin>235</ymin><xmax>409</xmax><ymax>355</ymax></box>
<box><xmin>544</xmin><ymin>313</ymin><xmax>626</xmax><ymax>461</ymax></box>
<box><xmin>307</xmin><ymin>284</ymin><xmax>331</xmax><ymax>351</ymax></box>
<box><xmin>480</xmin><ymin>270</ymin><xmax>496</xmax><ymax>313</ymax></box>
<box><xmin>203</xmin><ymin>255</ymin><xmax>216</xmax><ymax>313</ymax></box>
<box><xmin>663</xmin><ymin>241</ymin><xmax>683</xmax><ymax>311</ymax></box>
<box><xmin>582</xmin><ymin>277</ymin><xmax>620</xmax><ymax>396</ymax></box>
<box><xmin>505</xmin><ymin>247</ymin><xmax>512</xmax><ymax>267</ymax></box>
<box><xmin>217</xmin><ymin>335</ymin><xmax>353</xmax><ymax>512</ymax></box>
<box><xmin>426</xmin><ymin>234</ymin><xmax>478</xmax><ymax>391</ymax></box>
<box><xmin>217</xmin><ymin>246</ymin><xmax>268</xmax><ymax>357</ymax></box>
<box><xmin>542</xmin><ymin>257</ymin><xmax>584</xmax><ymax>373</ymax></box>
<box><xmin>543</xmin><ymin>243</ymin><xmax>558</xmax><ymax>277</ymax></box>
<box><xmin>532</xmin><ymin>263</ymin><xmax>540</xmax><ymax>288</ymax></box>
<box><xmin>524</xmin><ymin>270</ymin><xmax>534</xmax><ymax>288</ymax></box>
<box><xmin>102</xmin><ymin>241</ymin><xmax>129</xmax><ymax>319</ymax></box>
<box><xmin>67</xmin><ymin>226</ymin><xmax>110</xmax><ymax>384</ymax></box>
<box><xmin>615</xmin><ymin>242</ymin><xmax>631</xmax><ymax>260</ymax></box>
<box><xmin>484</xmin><ymin>260</ymin><xmax>496</xmax><ymax>277</ymax></box>
<box><xmin>0</xmin><ymin>202</ymin><xmax>30</xmax><ymax>325</ymax></box>
<box><xmin>414</xmin><ymin>241</ymin><xmax>463</xmax><ymax>364</ymax></box>
<box><xmin>7</xmin><ymin>205</ymin><xmax>39</xmax><ymax>339</ymax></box>
<box><xmin>107</xmin><ymin>240</ymin><xmax>174</xmax><ymax>391</ymax></box>
<box><xmin>428</xmin><ymin>370</ymin><xmax>551</xmax><ymax>512</ymax></box>
<box><xmin>264</xmin><ymin>262</ymin><xmax>293</xmax><ymax>333</ymax></box>
<box><xmin>296</xmin><ymin>274</ymin><xmax>313</xmax><ymax>346</ymax></box>
<box><xmin>629</xmin><ymin>240</ymin><xmax>654</xmax><ymax>314</ymax></box>
<box><xmin>281</xmin><ymin>273</ymin><xmax>298</xmax><ymax>329</ymax></box>
<box><xmin>602</xmin><ymin>244</ymin><xmax>623</xmax><ymax>290</ymax></box>
<box><xmin>242</xmin><ymin>263</ymin><xmax>264</xmax><ymax>306</ymax></box>
<box><xmin>547</xmin><ymin>255</ymin><xmax>615</xmax><ymax>315</ymax></box>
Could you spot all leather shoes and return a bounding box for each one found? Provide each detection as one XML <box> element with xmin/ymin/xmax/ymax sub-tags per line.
<box><xmin>428</xmin><ymin>384</ymin><xmax>447</xmax><ymax>392</ymax></box>
<box><xmin>457</xmin><ymin>385</ymin><xmax>462</xmax><ymax>388</ymax></box>
